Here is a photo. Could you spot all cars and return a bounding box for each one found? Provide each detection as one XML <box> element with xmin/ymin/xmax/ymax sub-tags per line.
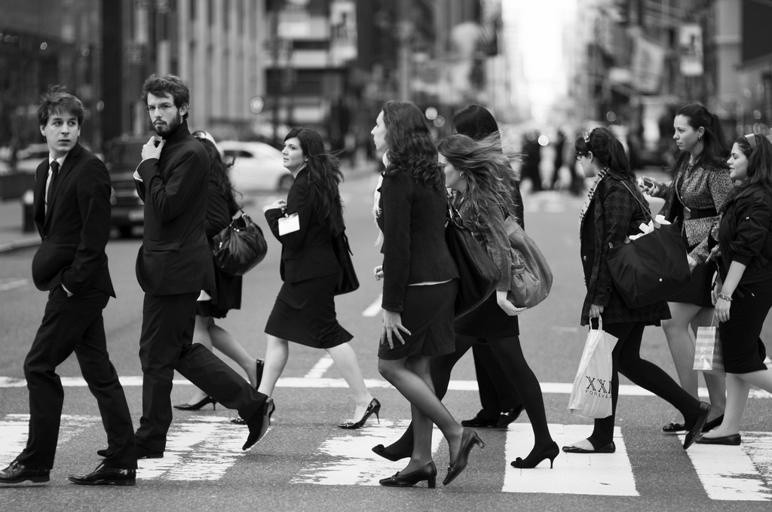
<box><xmin>213</xmin><ymin>139</ymin><xmax>293</xmax><ymax>194</ymax></box>
<box><xmin>0</xmin><ymin>141</ymin><xmax>91</xmax><ymax>172</ymax></box>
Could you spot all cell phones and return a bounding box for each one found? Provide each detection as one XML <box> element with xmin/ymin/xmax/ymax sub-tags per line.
<box><xmin>638</xmin><ymin>178</ymin><xmax>658</xmax><ymax>192</ymax></box>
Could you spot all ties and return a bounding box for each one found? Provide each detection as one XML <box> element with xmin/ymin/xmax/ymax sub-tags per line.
<box><xmin>47</xmin><ymin>161</ymin><xmax>59</xmax><ymax>213</ymax></box>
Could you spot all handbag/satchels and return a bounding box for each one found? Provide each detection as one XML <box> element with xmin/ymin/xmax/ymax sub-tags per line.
<box><xmin>692</xmin><ymin>326</ymin><xmax>725</xmax><ymax>376</ymax></box>
<box><xmin>607</xmin><ymin>222</ymin><xmax>692</xmax><ymax>310</ymax></box>
<box><xmin>445</xmin><ymin>215</ymin><xmax>553</xmax><ymax>321</ymax></box>
<box><xmin>213</xmin><ymin>214</ymin><xmax>267</xmax><ymax>277</ymax></box>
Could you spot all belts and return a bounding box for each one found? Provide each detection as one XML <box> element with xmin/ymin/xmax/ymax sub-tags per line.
<box><xmin>682</xmin><ymin>206</ymin><xmax>716</xmax><ymax>220</ymax></box>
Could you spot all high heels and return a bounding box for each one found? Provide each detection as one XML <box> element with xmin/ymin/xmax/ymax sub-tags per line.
<box><xmin>337</xmin><ymin>398</ymin><xmax>380</xmax><ymax>429</ymax></box>
<box><xmin>174</xmin><ymin>359</ymin><xmax>275</xmax><ymax>451</ymax></box>
<box><xmin>372</xmin><ymin>404</ymin><xmax>559</xmax><ymax>488</ymax></box>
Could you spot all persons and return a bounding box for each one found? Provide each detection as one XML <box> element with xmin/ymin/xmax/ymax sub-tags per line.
<box><xmin>371</xmin><ymin>100</ymin><xmax>559</xmax><ymax>486</ymax></box>
<box><xmin>563</xmin><ymin>126</ymin><xmax>711</xmax><ymax>453</ymax></box>
<box><xmin>641</xmin><ymin>103</ymin><xmax>772</xmax><ymax>446</ymax></box>
<box><xmin>229</xmin><ymin>128</ymin><xmax>381</xmax><ymax>429</ymax></box>
<box><xmin>0</xmin><ymin>87</ymin><xmax>139</xmax><ymax>486</ymax></box>
<box><xmin>95</xmin><ymin>73</ymin><xmax>279</xmax><ymax>455</ymax></box>
<box><xmin>518</xmin><ymin>130</ymin><xmax>587</xmax><ymax>196</ymax></box>
<box><xmin>174</xmin><ymin>130</ymin><xmax>263</xmax><ymax>415</ymax></box>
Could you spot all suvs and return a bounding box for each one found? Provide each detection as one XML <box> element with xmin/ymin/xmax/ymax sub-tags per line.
<box><xmin>100</xmin><ymin>136</ymin><xmax>150</xmax><ymax>234</ymax></box>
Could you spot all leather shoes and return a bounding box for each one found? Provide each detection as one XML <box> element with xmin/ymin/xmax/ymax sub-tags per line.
<box><xmin>68</xmin><ymin>440</ymin><xmax>163</xmax><ymax>486</ymax></box>
<box><xmin>563</xmin><ymin>442</ymin><xmax>615</xmax><ymax>453</ymax></box>
<box><xmin>663</xmin><ymin>401</ymin><xmax>741</xmax><ymax>449</ymax></box>
<box><xmin>0</xmin><ymin>460</ymin><xmax>50</xmax><ymax>482</ymax></box>
